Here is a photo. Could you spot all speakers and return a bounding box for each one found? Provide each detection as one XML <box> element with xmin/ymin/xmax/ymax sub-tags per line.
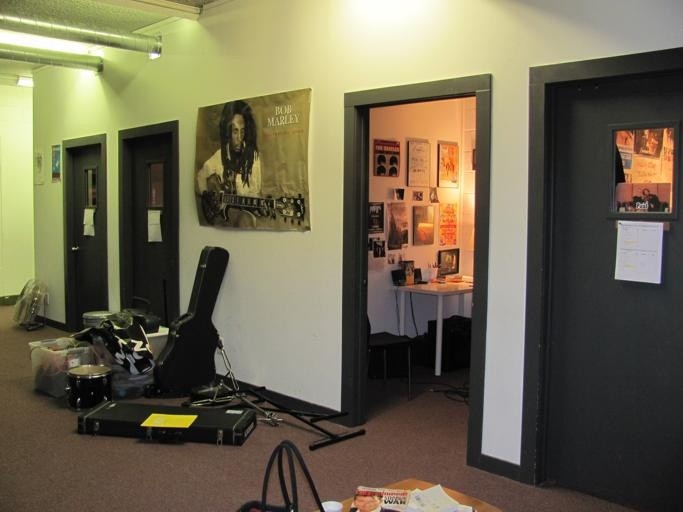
<box><xmin>414</xmin><ymin>268</ymin><xmax>422</xmax><ymax>284</ymax></box>
<box><xmin>391</xmin><ymin>269</ymin><xmax>406</xmax><ymax>286</ymax></box>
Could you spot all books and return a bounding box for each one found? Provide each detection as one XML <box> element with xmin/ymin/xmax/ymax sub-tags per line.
<box><xmin>350</xmin><ymin>485</ymin><xmax>411</xmax><ymax>512</ymax></box>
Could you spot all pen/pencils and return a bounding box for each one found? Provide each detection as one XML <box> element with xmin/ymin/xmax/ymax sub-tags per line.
<box><xmin>428</xmin><ymin>262</ymin><xmax>441</xmax><ymax>268</ymax></box>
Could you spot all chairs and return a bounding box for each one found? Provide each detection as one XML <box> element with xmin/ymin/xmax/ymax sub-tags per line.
<box><xmin>366</xmin><ymin>314</ymin><xmax>412</xmax><ymax>401</ymax></box>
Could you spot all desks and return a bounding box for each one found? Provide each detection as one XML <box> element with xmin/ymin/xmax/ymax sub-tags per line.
<box><xmin>387</xmin><ymin>276</ymin><xmax>473</xmax><ymax>376</ymax></box>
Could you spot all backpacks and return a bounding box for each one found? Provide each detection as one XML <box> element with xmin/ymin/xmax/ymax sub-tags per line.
<box><xmin>13</xmin><ymin>279</ymin><xmax>49</xmax><ymax>331</ymax></box>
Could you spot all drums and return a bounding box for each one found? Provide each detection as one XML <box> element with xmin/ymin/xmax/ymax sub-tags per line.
<box><xmin>64</xmin><ymin>363</ymin><xmax>113</xmax><ymax>411</ymax></box>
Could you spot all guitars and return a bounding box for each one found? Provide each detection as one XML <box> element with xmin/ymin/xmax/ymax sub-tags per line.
<box><xmin>201</xmin><ymin>174</ymin><xmax>305</xmax><ymax>227</ymax></box>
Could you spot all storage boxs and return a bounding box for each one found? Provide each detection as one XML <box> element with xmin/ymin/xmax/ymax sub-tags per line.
<box><xmin>28</xmin><ymin>325</ymin><xmax>170</xmax><ymax>399</ymax></box>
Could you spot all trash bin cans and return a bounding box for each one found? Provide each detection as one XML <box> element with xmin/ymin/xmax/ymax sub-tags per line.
<box><xmin>82</xmin><ymin>312</ymin><xmax>113</xmax><ymax>328</ymax></box>
<box><xmin>427</xmin><ymin>318</ymin><xmax>471</xmax><ymax>370</ymax></box>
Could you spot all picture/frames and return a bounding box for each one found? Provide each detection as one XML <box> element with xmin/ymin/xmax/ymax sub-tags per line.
<box><xmin>368</xmin><ymin>202</ymin><xmax>384</xmax><ymax>234</ymax></box>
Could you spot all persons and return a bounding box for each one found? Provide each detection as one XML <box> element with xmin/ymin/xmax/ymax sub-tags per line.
<box><xmin>376</xmin><ymin>155</ymin><xmax>400</xmax><ymax>176</ymax></box>
<box><xmin>642</xmin><ymin>189</ymin><xmax>660</xmax><ymax>211</ymax></box>
<box><xmin>196</xmin><ymin>100</ymin><xmax>261</xmax><ymax>229</ymax></box>
<box><xmin>350</xmin><ymin>491</ymin><xmax>400</xmax><ymax>512</ymax></box>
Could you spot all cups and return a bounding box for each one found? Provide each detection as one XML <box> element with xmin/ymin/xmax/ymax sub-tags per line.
<box><xmin>427</xmin><ymin>268</ymin><xmax>437</xmax><ymax>280</ymax></box>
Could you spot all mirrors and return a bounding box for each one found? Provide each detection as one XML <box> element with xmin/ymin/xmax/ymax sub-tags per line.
<box><xmin>608</xmin><ymin>122</ymin><xmax>679</xmax><ymax>220</ymax></box>
